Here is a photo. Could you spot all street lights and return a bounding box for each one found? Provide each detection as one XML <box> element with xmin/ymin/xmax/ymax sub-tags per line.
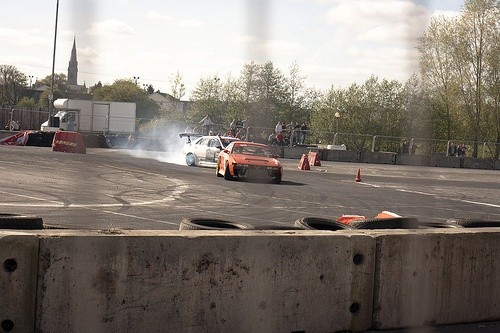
<box><xmin>134</xmin><ymin>77</ymin><xmax>140</xmax><ymax>87</ymax></box>
<box><xmin>335</xmin><ymin>112</ymin><xmax>340</xmax><ymax>133</ymax></box>
<box><xmin>143</xmin><ymin>83</ymin><xmax>148</xmax><ymax>92</ymax></box>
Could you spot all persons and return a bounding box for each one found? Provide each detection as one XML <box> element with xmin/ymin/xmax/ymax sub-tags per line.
<box><xmin>5</xmin><ymin>119</ymin><xmax>21</xmax><ymax>131</ymax></box>
<box><xmin>396</xmin><ymin>137</ymin><xmax>419</xmax><ymax>158</ymax></box>
<box><xmin>448</xmin><ymin>143</ymin><xmax>468</xmax><ymax>157</ymax></box>
<box><xmin>333</xmin><ymin>132</ymin><xmax>338</xmax><ymax>144</ymax></box>
<box><xmin>268</xmin><ymin>121</ymin><xmax>307</xmax><ymax>145</ymax></box>
<box><xmin>185</xmin><ymin>114</ymin><xmax>268</xmax><ymax>142</ymax></box>
<box><xmin>314</xmin><ymin>138</ymin><xmax>319</xmax><ymax>144</ymax></box>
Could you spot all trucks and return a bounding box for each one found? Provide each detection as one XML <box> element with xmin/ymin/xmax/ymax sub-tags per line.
<box><xmin>41</xmin><ymin>98</ymin><xmax>137</xmax><ymax>133</ymax></box>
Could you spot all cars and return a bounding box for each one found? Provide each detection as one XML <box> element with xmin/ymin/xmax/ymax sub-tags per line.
<box><xmin>178</xmin><ymin>132</ymin><xmax>240</xmax><ymax>167</ymax></box>
<box><xmin>215</xmin><ymin>141</ymin><xmax>283</xmax><ymax>185</ymax></box>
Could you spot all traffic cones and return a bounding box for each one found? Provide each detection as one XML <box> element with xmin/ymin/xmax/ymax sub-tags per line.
<box><xmin>355</xmin><ymin>168</ymin><xmax>362</xmax><ymax>182</ymax></box>
